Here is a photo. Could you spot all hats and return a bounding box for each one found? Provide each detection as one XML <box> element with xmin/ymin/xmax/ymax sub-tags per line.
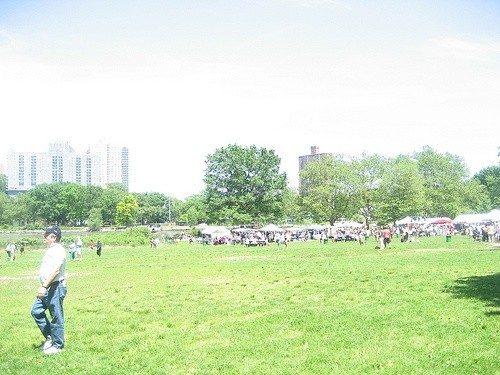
<box><xmin>45</xmin><ymin>225</ymin><xmax>62</xmax><ymax>238</ymax></box>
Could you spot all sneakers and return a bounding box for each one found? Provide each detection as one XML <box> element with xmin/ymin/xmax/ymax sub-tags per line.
<box><xmin>39</xmin><ymin>335</ymin><xmax>53</xmax><ymax>350</ymax></box>
<box><xmin>43</xmin><ymin>346</ymin><xmax>63</xmax><ymax>356</ymax></box>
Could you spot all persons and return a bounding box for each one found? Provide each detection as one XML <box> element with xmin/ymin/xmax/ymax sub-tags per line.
<box><xmin>69</xmin><ymin>239</ymin><xmax>76</xmax><ymax>262</ymax></box>
<box><xmin>150</xmin><ymin>236</ymin><xmax>160</xmax><ymax>248</ymax></box>
<box><xmin>89</xmin><ymin>239</ymin><xmax>94</xmax><ymax>252</ymax></box>
<box><xmin>5</xmin><ymin>240</ymin><xmax>26</xmax><ymax>261</ymax></box>
<box><xmin>97</xmin><ymin>239</ymin><xmax>102</xmax><ymax>257</ymax></box>
<box><xmin>75</xmin><ymin>235</ymin><xmax>83</xmax><ymax>261</ymax></box>
<box><xmin>180</xmin><ymin>222</ymin><xmax>500</xmax><ymax>250</ymax></box>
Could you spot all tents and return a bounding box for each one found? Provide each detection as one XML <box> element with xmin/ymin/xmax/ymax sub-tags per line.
<box><xmin>395</xmin><ymin>208</ymin><xmax>500</xmax><ymax>224</ymax></box>
<box><xmin>195</xmin><ymin>222</ymin><xmax>363</xmax><ymax>238</ymax></box>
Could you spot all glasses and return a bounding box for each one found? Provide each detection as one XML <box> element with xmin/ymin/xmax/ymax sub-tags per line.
<box><xmin>43</xmin><ymin>233</ymin><xmax>51</xmax><ymax>238</ymax></box>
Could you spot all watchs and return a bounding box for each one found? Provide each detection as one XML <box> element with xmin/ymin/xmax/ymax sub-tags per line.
<box><xmin>31</xmin><ymin>225</ymin><xmax>68</xmax><ymax>356</ymax></box>
<box><xmin>42</xmin><ymin>285</ymin><xmax>49</xmax><ymax>290</ymax></box>
